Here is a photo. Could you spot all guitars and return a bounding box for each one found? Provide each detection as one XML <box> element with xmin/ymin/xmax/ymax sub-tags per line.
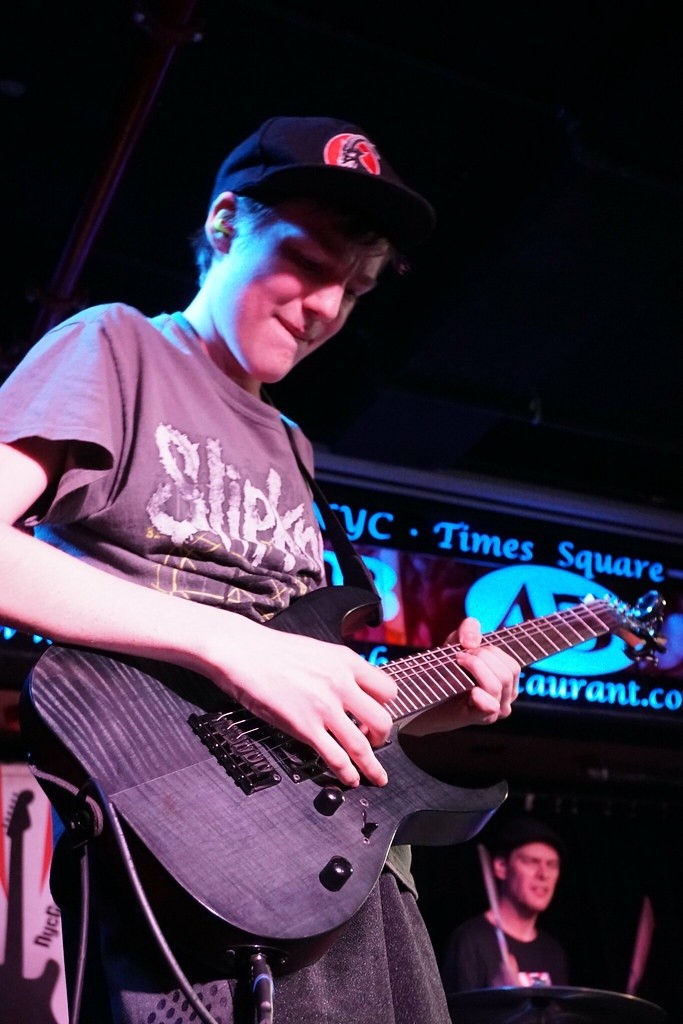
<box><xmin>21</xmin><ymin>587</ymin><xmax>668</xmax><ymax>973</ymax></box>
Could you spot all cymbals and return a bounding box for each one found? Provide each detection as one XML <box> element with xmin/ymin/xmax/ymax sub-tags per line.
<box><xmin>447</xmin><ymin>985</ymin><xmax>673</xmax><ymax>1024</ymax></box>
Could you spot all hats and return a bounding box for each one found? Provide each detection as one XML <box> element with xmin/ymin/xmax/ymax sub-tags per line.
<box><xmin>213</xmin><ymin>114</ymin><xmax>432</xmax><ymax>234</ymax></box>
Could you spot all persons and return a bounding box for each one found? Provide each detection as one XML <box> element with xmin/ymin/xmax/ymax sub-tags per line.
<box><xmin>0</xmin><ymin>115</ymin><xmax>523</xmax><ymax>1024</ymax></box>
<box><xmin>441</xmin><ymin>818</ymin><xmax>572</xmax><ymax>1024</ymax></box>
